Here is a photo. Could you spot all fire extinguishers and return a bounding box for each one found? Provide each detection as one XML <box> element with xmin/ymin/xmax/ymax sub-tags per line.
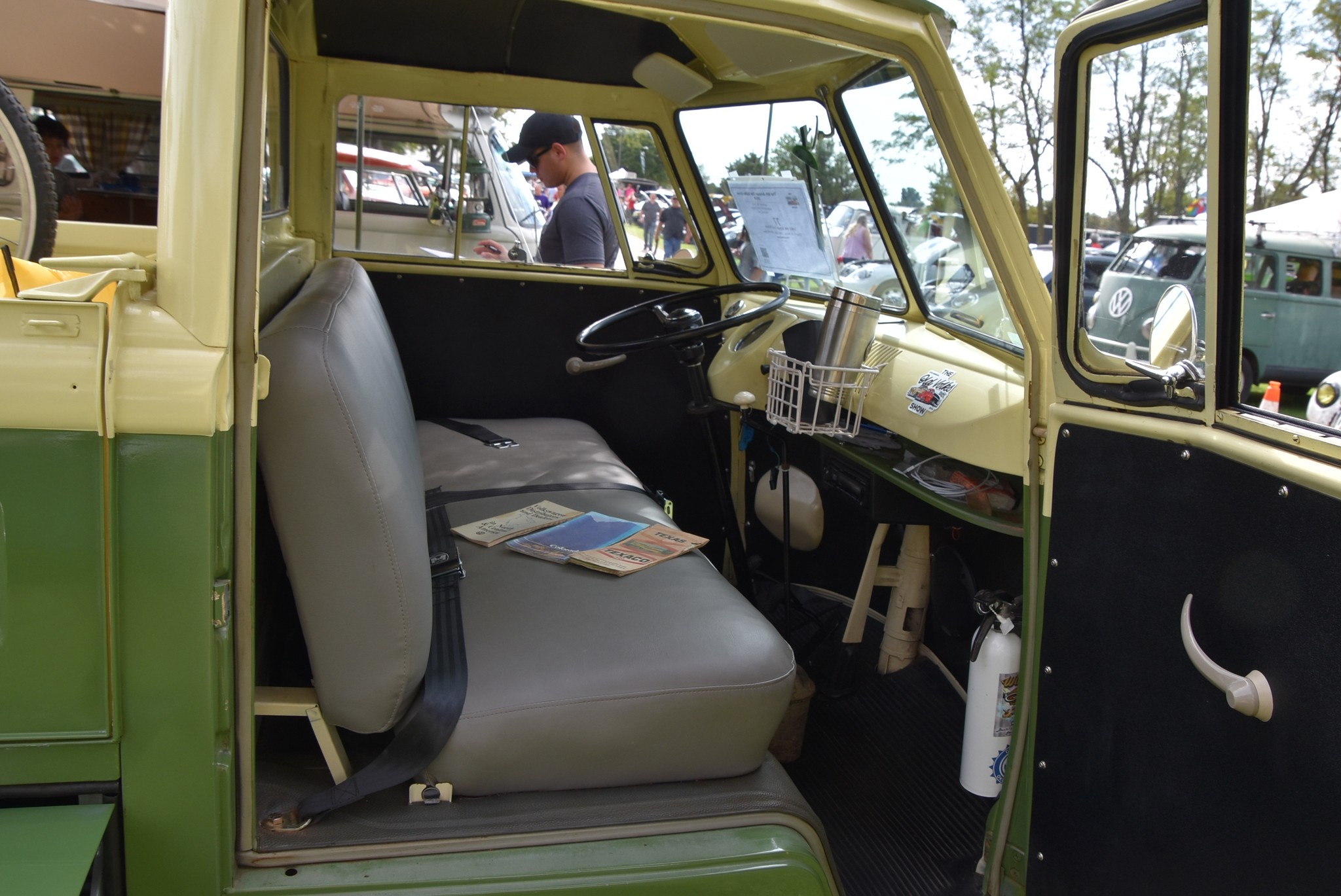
<box><xmin>958</xmin><ymin>589</ymin><xmax>1022</xmax><ymax>798</ymax></box>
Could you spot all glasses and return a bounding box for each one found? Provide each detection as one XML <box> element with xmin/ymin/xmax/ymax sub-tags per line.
<box><xmin>526</xmin><ymin>148</ymin><xmax>551</xmax><ymax>168</ymax></box>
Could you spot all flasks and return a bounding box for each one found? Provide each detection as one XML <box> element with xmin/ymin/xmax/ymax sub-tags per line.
<box><xmin>807</xmin><ymin>285</ymin><xmax>884</xmax><ymax>405</ymax></box>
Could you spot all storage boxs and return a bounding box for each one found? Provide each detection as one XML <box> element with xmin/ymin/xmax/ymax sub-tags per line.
<box><xmin>768</xmin><ymin>662</ymin><xmax>816</xmax><ymax>764</ymax></box>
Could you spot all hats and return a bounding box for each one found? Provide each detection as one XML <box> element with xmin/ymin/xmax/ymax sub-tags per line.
<box><xmin>672</xmin><ymin>194</ymin><xmax>678</xmax><ymax>199</ymax></box>
<box><xmin>501</xmin><ymin>111</ymin><xmax>584</xmax><ymax>164</ymax></box>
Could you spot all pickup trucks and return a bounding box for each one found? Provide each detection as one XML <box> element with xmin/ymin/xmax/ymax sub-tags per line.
<box><xmin>2</xmin><ymin>0</ymin><xmax>1341</xmax><ymax>896</ymax></box>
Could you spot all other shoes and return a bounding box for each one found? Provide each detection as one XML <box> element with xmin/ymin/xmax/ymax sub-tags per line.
<box><xmin>644</xmin><ymin>247</ymin><xmax>647</xmax><ymax>250</ymax></box>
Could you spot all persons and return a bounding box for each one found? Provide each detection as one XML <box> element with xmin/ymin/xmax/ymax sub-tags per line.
<box><xmin>32</xmin><ymin>115</ymin><xmax>88</xmax><ymax>174</ymax></box>
<box><xmin>473</xmin><ymin>112</ymin><xmax>625</xmax><ymax>271</ymax></box>
<box><xmin>624</xmin><ymin>183</ymin><xmax>635</xmax><ymax>207</ymax></box>
<box><xmin>553</xmin><ymin>185</ymin><xmax>566</xmax><ymax>202</ymax></box>
<box><xmin>898</xmin><ymin>211</ymin><xmax>911</xmax><ymax>234</ymax></box>
<box><xmin>624</xmin><ymin>192</ymin><xmax>637</xmax><ymax>224</ymax></box>
<box><xmin>729</xmin><ymin>225</ymin><xmax>769</xmax><ymax>282</ymax></box>
<box><xmin>638</xmin><ymin>193</ymin><xmax>662</xmax><ymax>251</ymax></box>
<box><xmin>1088</xmin><ymin>235</ymin><xmax>1102</xmax><ymax>249</ymax></box>
<box><xmin>532</xmin><ymin>182</ymin><xmax>551</xmax><ymax>219</ymax></box>
<box><xmin>917</xmin><ymin>214</ymin><xmax>930</xmax><ymax>237</ymax></box>
<box><xmin>842</xmin><ymin>212</ymin><xmax>873</xmax><ymax>265</ymax></box>
<box><xmin>655</xmin><ymin>194</ymin><xmax>692</xmax><ymax>261</ymax></box>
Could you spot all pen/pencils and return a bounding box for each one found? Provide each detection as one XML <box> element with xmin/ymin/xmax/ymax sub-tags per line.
<box><xmin>484</xmin><ymin>244</ymin><xmax>501</xmax><ymax>254</ymax></box>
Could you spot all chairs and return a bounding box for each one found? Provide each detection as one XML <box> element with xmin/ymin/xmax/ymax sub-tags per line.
<box><xmin>1286</xmin><ymin>263</ymin><xmax>1321</xmax><ymax>296</ymax></box>
<box><xmin>336</xmin><ymin>191</ymin><xmax>353</xmax><ymax>212</ymax></box>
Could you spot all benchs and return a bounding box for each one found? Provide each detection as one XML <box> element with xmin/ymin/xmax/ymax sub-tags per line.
<box><xmin>259</xmin><ymin>255</ymin><xmax>799</xmax><ymax>798</ymax></box>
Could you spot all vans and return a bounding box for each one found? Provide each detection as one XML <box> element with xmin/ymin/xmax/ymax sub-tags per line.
<box><xmin>1085</xmin><ymin>223</ymin><xmax>1341</xmax><ymax>412</ymax></box>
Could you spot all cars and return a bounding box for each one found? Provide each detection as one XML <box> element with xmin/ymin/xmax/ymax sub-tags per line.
<box><xmin>260</xmin><ymin>157</ymin><xmax>1126</xmax><ymax>359</ymax></box>
<box><xmin>1306</xmin><ymin>368</ymin><xmax>1341</xmax><ymax>432</ymax></box>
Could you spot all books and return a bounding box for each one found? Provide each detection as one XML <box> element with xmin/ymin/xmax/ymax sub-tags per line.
<box><xmin>504</xmin><ymin>510</ymin><xmax>651</xmax><ymax>566</ymax></box>
<box><xmin>449</xmin><ymin>499</ymin><xmax>586</xmax><ymax>550</ymax></box>
<box><xmin>418</xmin><ymin>247</ymin><xmax>467</xmax><ymax>259</ymax></box>
<box><xmin>567</xmin><ymin>522</ymin><xmax>711</xmax><ymax>578</ymax></box>
